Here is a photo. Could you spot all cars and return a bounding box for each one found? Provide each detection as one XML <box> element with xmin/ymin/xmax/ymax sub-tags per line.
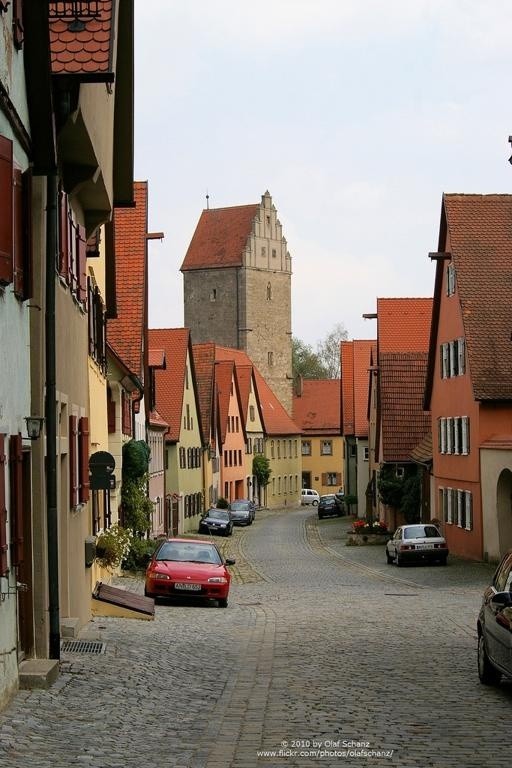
<box><xmin>142</xmin><ymin>537</ymin><xmax>237</xmax><ymax>608</ymax></box>
<box><xmin>196</xmin><ymin>506</ymin><xmax>234</xmax><ymax>537</ymax></box>
<box><xmin>227</xmin><ymin>502</ymin><xmax>254</xmax><ymax>526</ymax></box>
<box><xmin>317</xmin><ymin>493</ymin><xmax>344</xmax><ymax>520</ymax></box>
<box><xmin>474</xmin><ymin>546</ymin><xmax>512</xmax><ymax>690</ymax></box>
<box><xmin>384</xmin><ymin>522</ymin><xmax>450</xmax><ymax>569</ymax></box>
<box><xmin>235</xmin><ymin>499</ymin><xmax>256</xmax><ymax>519</ymax></box>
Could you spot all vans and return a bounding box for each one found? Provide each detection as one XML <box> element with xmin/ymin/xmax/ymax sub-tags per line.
<box><xmin>301</xmin><ymin>489</ymin><xmax>320</xmax><ymax>507</ymax></box>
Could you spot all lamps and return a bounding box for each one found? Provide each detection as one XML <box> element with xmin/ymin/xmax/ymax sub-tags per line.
<box><xmin>19</xmin><ymin>415</ymin><xmax>46</xmax><ymax>441</ymax></box>
